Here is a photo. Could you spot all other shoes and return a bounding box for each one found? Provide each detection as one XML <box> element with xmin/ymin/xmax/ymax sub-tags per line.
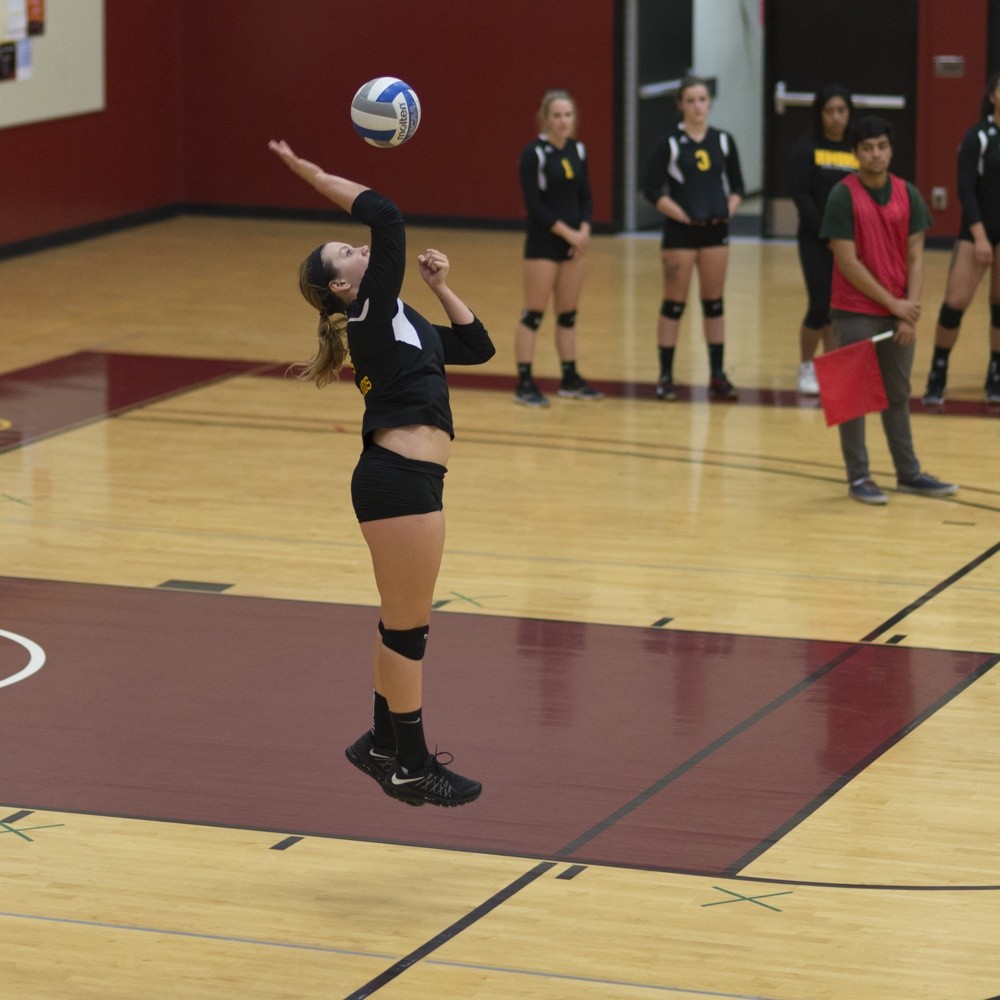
<box><xmin>710</xmin><ymin>372</ymin><xmax>737</xmax><ymax>400</ymax></box>
<box><xmin>558</xmin><ymin>370</ymin><xmax>607</xmax><ymax>400</ymax></box>
<box><xmin>986</xmin><ymin>374</ymin><xmax>1000</xmax><ymax>404</ymax></box>
<box><xmin>799</xmin><ymin>360</ymin><xmax>820</xmax><ymax>395</ymax></box>
<box><xmin>514</xmin><ymin>376</ymin><xmax>551</xmax><ymax>408</ymax></box>
<box><xmin>657</xmin><ymin>375</ymin><xmax>677</xmax><ymax>401</ymax></box>
<box><xmin>923</xmin><ymin>371</ymin><xmax>946</xmax><ymax>404</ymax></box>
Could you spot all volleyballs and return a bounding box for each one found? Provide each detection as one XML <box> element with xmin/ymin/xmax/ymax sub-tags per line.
<box><xmin>350</xmin><ymin>77</ymin><xmax>422</xmax><ymax>148</ymax></box>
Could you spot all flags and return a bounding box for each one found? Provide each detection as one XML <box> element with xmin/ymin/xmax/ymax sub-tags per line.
<box><xmin>814</xmin><ymin>335</ymin><xmax>889</xmax><ymax>427</ymax></box>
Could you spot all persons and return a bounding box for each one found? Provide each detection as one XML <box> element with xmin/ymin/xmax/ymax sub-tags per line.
<box><xmin>921</xmin><ymin>72</ymin><xmax>999</xmax><ymax>409</ymax></box>
<box><xmin>269</xmin><ymin>141</ymin><xmax>498</xmax><ymax>809</ymax></box>
<box><xmin>790</xmin><ymin>82</ymin><xmax>866</xmax><ymax>393</ymax></box>
<box><xmin>640</xmin><ymin>76</ymin><xmax>744</xmax><ymax>403</ymax></box>
<box><xmin>513</xmin><ymin>87</ymin><xmax>603</xmax><ymax>409</ymax></box>
<box><xmin>817</xmin><ymin>116</ymin><xmax>958</xmax><ymax>506</ymax></box>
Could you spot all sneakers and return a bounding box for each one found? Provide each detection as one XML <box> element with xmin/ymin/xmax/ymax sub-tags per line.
<box><xmin>383</xmin><ymin>744</ymin><xmax>482</xmax><ymax>807</ymax></box>
<box><xmin>344</xmin><ymin>728</ymin><xmax>426</xmax><ymax>805</ymax></box>
<box><xmin>897</xmin><ymin>471</ymin><xmax>959</xmax><ymax>497</ymax></box>
<box><xmin>848</xmin><ymin>478</ymin><xmax>887</xmax><ymax>504</ymax></box>
